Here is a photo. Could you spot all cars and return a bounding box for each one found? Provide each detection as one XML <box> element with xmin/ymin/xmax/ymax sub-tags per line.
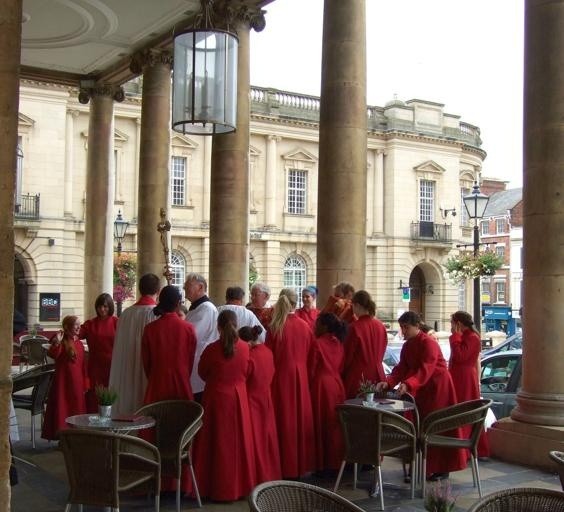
<box><xmin>379</xmin><ymin>330</ymin><xmax>523</xmax><ymax>420</ymax></box>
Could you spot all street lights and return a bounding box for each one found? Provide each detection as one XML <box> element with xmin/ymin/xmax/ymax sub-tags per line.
<box><xmin>455</xmin><ymin>181</ymin><xmax>497</xmax><ymax>339</ymax></box>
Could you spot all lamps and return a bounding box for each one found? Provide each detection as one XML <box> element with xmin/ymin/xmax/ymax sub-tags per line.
<box><xmin>444</xmin><ymin>208</ymin><xmax>456</xmax><ymax>218</ymax></box>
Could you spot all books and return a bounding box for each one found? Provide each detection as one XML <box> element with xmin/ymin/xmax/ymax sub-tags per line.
<box><xmin>111</xmin><ymin>414</ymin><xmax>143</xmax><ymax>422</ymax></box>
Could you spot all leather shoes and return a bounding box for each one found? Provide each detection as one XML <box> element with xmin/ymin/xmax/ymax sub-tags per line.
<box><xmin>430</xmin><ymin>472</ymin><xmax>449</xmax><ymax>481</ymax></box>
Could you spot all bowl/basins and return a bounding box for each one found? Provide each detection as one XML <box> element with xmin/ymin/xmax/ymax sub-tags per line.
<box><xmin>88</xmin><ymin>416</ymin><xmax>110</xmax><ymax>424</ymax></box>
<box><xmin>361</xmin><ymin>400</ymin><xmax>380</xmax><ymax>408</ymax></box>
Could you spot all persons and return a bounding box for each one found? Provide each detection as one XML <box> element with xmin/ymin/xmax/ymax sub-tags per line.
<box><xmin>50</xmin><ymin>293</ymin><xmax>119</xmax><ymax>407</ymax></box>
<box><xmin>448</xmin><ymin>311</ymin><xmax>489</xmax><ymax>457</ymax></box>
<box><xmin>108</xmin><ymin>274</ymin><xmax>161</xmax><ymax>416</ymax></box>
<box><xmin>40</xmin><ymin>315</ymin><xmax>90</xmax><ymax>441</ymax></box>
<box><xmin>375</xmin><ymin>310</ymin><xmax>467</xmax><ymax>481</ymax></box>
<box><xmin>421</xmin><ymin>324</ymin><xmax>438</xmax><ymax>343</ymax></box>
<box><xmin>138</xmin><ymin>274</ymin><xmax>386</xmax><ymax>503</ymax></box>
